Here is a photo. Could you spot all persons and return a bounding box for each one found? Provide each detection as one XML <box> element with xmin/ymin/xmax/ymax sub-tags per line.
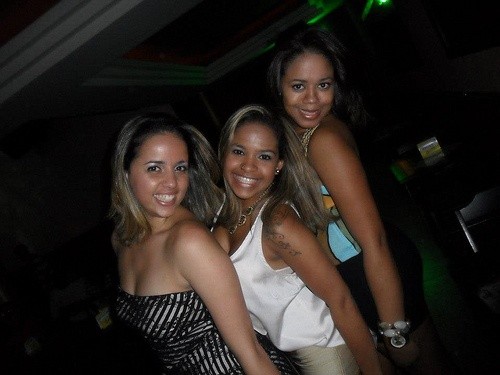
<box><xmin>207</xmin><ymin>105</ymin><xmax>391</xmax><ymax>375</ymax></box>
<box><xmin>265</xmin><ymin>23</ymin><xmax>424</xmax><ymax>375</ymax></box>
<box><xmin>110</xmin><ymin>113</ymin><xmax>301</xmax><ymax>375</ymax></box>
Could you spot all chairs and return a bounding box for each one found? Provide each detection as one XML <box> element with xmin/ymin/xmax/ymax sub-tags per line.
<box><xmin>454</xmin><ymin>189</ymin><xmax>499</xmax><ymax>256</ymax></box>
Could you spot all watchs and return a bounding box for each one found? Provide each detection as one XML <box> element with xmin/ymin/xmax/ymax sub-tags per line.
<box><xmin>382</xmin><ymin>322</ymin><xmax>412</xmax><ymax>351</ymax></box>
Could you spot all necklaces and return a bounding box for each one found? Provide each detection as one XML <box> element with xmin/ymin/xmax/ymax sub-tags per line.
<box><xmin>229</xmin><ymin>182</ymin><xmax>274</xmax><ymax>235</ymax></box>
<box><xmin>295</xmin><ymin>123</ymin><xmax>321</xmax><ymax>156</ymax></box>
